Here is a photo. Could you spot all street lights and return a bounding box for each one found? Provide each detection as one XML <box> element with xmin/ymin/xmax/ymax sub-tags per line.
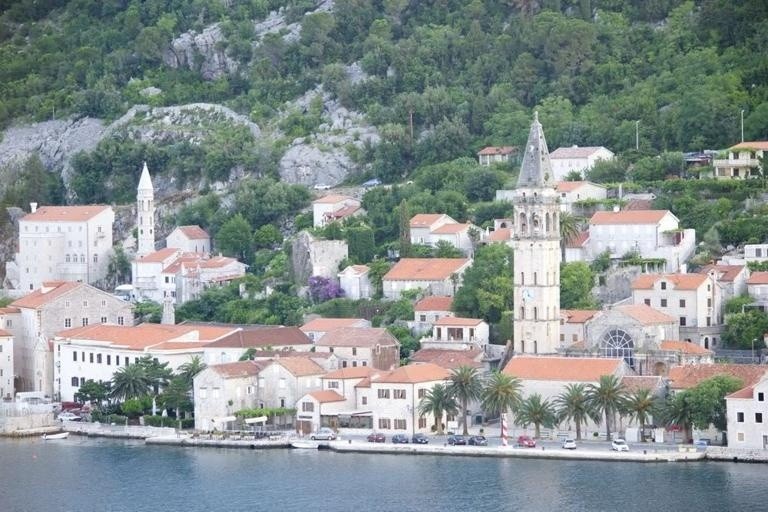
<box><xmin>752</xmin><ymin>338</ymin><xmax>758</xmax><ymax>363</ymax></box>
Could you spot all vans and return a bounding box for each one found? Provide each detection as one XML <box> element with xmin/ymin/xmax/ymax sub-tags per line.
<box><xmin>16</xmin><ymin>391</ymin><xmax>53</xmax><ymax>403</ymax></box>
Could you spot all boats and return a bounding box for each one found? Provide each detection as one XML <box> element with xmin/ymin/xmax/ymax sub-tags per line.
<box><xmin>40</xmin><ymin>432</ymin><xmax>69</xmax><ymax>440</ymax></box>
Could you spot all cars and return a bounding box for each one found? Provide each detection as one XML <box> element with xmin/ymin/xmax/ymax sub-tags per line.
<box><xmin>311</xmin><ymin>427</ymin><xmax>337</xmax><ymax>440</ymax></box>
<box><xmin>518</xmin><ymin>436</ymin><xmax>536</xmax><ymax>448</ymax></box>
<box><xmin>612</xmin><ymin>439</ymin><xmax>629</xmax><ymax>450</ymax></box>
<box><xmin>447</xmin><ymin>435</ymin><xmax>488</xmax><ymax>446</ymax></box>
<box><xmin>562</xmin><ymin>439</ymin><xmax>576</xmax><ymax>448</ymax></box>
<box><xmin>695</xmin><ymin>439</ymin><xmax>711</xmax><ymax>445</ymax></box>
<box><xmin>20</xmin><ymin>396</ymin><xmax>90</xmax><ymax>421</ymax></box>
<box><xmin>412</xmin><ymin>432</ymin><xmax>429</xmax><ymax>443</ymax></box>
<box><xmin>190</xmin><ymin>431</ymin><xmax>286</xmax><ymax>440</ymax></box>
<box><xmin>367</xmin><ymin>433</ymin><xmax>386</xmax><ymax>442</ymax></box>
<box><xmin>392</xmin><ymin>433</ymin><xmax>408</xmax><ymax>443</ymax></box>
